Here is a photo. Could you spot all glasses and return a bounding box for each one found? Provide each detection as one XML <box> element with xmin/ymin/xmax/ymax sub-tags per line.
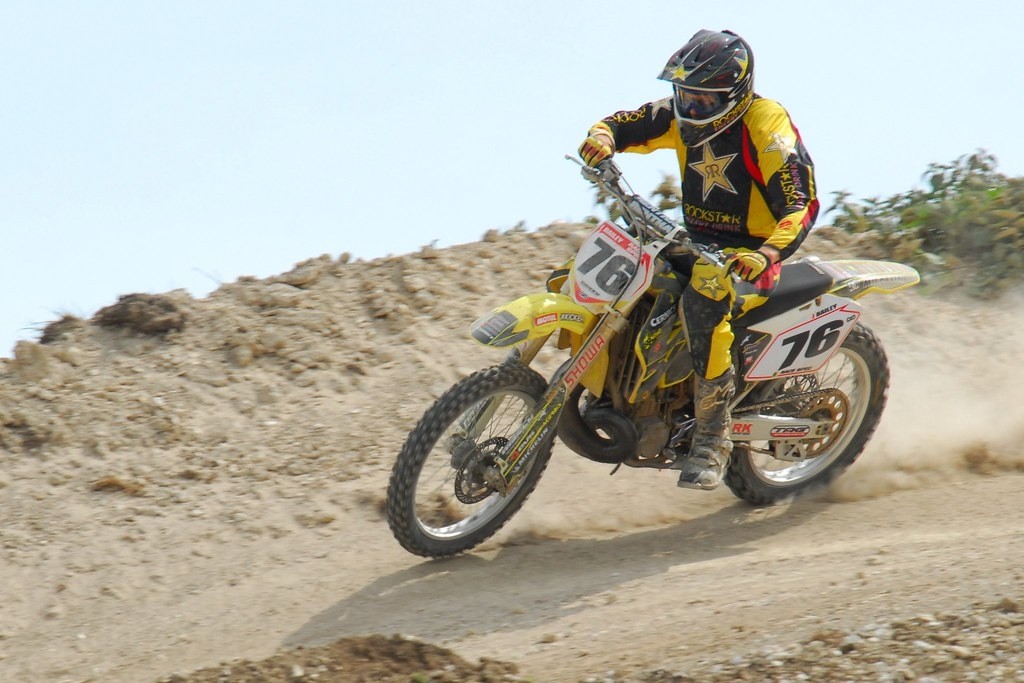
<box><xmin>675</xmin><ymin>86</ymin><xmax>727</xmax><ymax>116</ymax></box>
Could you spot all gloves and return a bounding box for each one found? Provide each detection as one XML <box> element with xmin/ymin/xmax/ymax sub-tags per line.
<box><xmin>578</xmin><ymin>136</ymin><xmax>613</xmax><ymax>168</ymax></box>
<box><xmin>722</xmin><ymin>251</ymin><xmax>771</xmax><ymax>285</ymax></box>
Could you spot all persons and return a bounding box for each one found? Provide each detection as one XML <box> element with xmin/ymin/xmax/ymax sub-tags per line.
<box><xmin>578</xmin><ymin>29</ymin><xmax>820</xmax><ymax>491</ymax></box>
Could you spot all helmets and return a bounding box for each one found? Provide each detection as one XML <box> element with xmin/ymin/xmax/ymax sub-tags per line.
<box><xmin>655</xmin><ymin>29</ymin><xmax>755</xmax><ymax>148</ymax></box>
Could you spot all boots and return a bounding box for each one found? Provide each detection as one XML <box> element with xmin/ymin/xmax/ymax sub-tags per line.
<box><xmin>677</xmin><ymin>365</ymin><xmax>737</xmax><ymax>489</ymax></box>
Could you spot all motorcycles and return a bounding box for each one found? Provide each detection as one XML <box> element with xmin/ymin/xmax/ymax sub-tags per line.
<box><xmin>385</xmin><ymin>153</ymin><xmax>921</xmax><ymax>559</ymax></box>
